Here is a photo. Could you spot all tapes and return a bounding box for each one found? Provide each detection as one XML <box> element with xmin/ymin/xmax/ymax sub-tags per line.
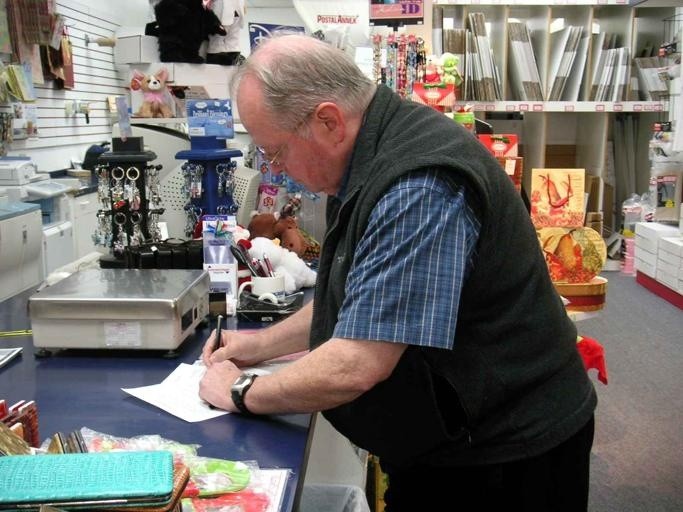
<box><xmin>258</xmin><ymin>292</ymin><xmax>278</xmax><ymax>304</ymax></box>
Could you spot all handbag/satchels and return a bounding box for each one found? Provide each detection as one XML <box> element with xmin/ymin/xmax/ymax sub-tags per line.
<box><xmin>530</xmin><ymin>168</ymin><xmax>585</xmax><ymax>228</ymax></box>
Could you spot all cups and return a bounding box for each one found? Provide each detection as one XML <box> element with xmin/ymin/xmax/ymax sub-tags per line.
<box><xmin>238</xmin><ymin>275</ymin><xmax>285</xmax><ymax>306</ymax></box>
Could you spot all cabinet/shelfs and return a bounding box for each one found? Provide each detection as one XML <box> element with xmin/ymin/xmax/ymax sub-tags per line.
<box><xmin>431</xmin><ymin>0</ymin><xmax>682</xmax><ymax>270</ymax></box>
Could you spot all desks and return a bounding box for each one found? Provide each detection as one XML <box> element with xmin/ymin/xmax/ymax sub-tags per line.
<box><xmin>0</xmin><ymin>258</ymin><xmax>321</xmax><ymax>512</ymax></box>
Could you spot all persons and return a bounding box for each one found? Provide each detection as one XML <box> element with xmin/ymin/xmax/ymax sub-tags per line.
<box><xmin>198</xmin><ymin>31</ymin><xmax>598</xmax><ymax>512</ymax></box>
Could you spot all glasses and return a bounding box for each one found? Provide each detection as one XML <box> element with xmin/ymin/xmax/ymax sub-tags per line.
<box><xmin>244</xmin><ymin>112</ymin><xmax>318</xmax><ymax>166</ymax></box>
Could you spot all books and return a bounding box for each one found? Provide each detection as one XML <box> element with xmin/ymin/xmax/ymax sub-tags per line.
<box><xmin>433</xmin><ymin>6</ymin><xmax>669</xmax><ymax>101</ymax></box>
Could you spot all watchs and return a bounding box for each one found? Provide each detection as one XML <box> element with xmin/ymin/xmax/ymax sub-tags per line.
<box><xmin>230</xmin><ymin>371</ymin><xmax>257</xmax><ymax>418</ymax></box>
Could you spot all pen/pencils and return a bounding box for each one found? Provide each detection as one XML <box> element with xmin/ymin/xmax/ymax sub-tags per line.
<box><xmin>214</xmin><ymin>315</ymin><xmax>224</xmax><ymax>350</ymax></box>
<box><xmin>230</xmin><ymin>242</ymin><xmax>274</xmax><ymax>277</ymax></box>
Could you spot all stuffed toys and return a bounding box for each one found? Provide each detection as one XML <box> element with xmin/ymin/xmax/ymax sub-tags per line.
<box><xmin>233</xmin><ymin>204</ymin><xmax>317</xmax><ymax>295</ymax></box>
<box><xmin>130</xmin><ymin>66</ymin><xmax>177</xmax><ymax>118</ymax></box>
